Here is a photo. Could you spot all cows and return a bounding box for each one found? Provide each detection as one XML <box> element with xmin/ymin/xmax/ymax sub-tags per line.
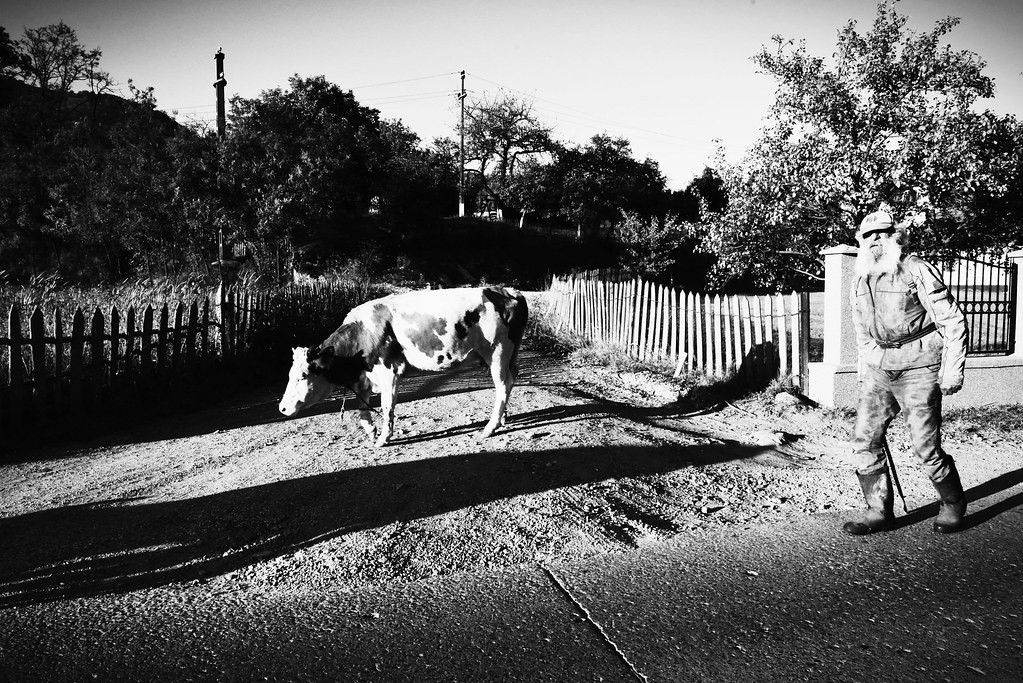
<box><xmin>278</xmin><ymin>286</ymin><xmax>530</xmax><ymax>448</ymax></box>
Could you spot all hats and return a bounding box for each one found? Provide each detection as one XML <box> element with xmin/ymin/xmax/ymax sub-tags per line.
<box><xmin>858</xmin><ymin>211</ymin><xmax>894</xmax><ymax>235</ymax></box>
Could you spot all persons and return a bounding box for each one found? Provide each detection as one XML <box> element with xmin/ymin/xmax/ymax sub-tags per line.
<box><xmin>841</xmin><ymin>207</ymin><xmax>970</xmax><ymax>537</ymax></box>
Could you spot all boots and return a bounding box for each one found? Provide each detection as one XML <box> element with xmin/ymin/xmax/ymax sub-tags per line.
<box><xmin>929</xmin><ymin>455</ymin><xmax>966</xmax><ymax>533</ymax></box>
<box><xmin>843</xmin><ymin>470</ymin><xmax>895</xmax><ymax>534</ymax></box>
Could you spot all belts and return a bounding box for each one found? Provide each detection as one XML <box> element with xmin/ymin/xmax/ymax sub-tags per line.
<box><xmin>876</xmin><ymin>322</ymin><xmax>937</xmax><ymax>349</ymax></box>
